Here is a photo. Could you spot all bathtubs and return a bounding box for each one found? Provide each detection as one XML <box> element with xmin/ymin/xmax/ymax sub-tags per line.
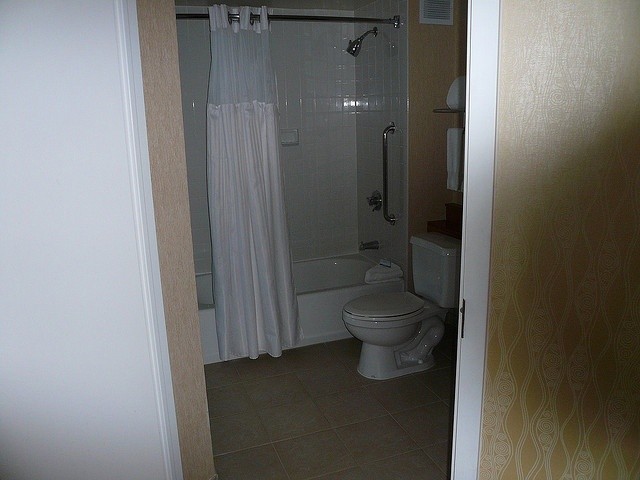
<box><xmin>195</xmin><ymin>254</ymin><xmax>404</xmax><ymax>365</ymax></box>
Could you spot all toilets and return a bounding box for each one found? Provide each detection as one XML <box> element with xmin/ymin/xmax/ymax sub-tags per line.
<box><xmin>342</xmin><ymin>231</ymin><xmax>461</xmax><ymax>381</ymax></box>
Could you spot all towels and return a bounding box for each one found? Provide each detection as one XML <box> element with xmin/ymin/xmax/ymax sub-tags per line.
<box><xmin>363</xmin><ymin>261</ymin><xmax>404</xmax><ymax>285</ymax></box>
<box><xmin>445</xmin><ymin>128</ymin><xmax>463</xmax><ymax>193</ymax></box>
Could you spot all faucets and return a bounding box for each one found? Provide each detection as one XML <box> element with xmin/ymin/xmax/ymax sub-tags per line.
<box><xmin>359</xmin><ymin>240</ymin><xmax>379</xmax><ymax>250</ymax></box>
